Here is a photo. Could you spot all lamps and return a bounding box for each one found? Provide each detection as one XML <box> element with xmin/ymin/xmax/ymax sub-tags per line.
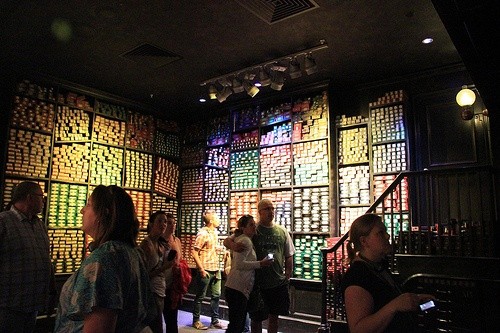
<box><xmin>288</xmin><ymin>56</ymin><xmax>303</xmax><ymax>80</ymax></box>
<box><xmin>209</xmin><ymin>62</ymin><xmax>287</xmax><ymax>103</ymax></box>
<box><xmin>303</xmin><ymin>53</ymin><xmax>318</xmax><ymax>76</ymax></box>
<box><xmin>456</xmin><ymin>84</ymin><xmax>482</xmax><ymax>120</ymax></box>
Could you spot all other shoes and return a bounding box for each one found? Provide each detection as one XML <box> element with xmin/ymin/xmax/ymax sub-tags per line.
<box><xmin>213</xmin><ymin>322</ymin><xmax>228</xmax><ymax>329</ymax></box>
<box><xmin>192</xmin><ymin>320</ymin><xmax>208</xmax><ymax>330</ymax></box>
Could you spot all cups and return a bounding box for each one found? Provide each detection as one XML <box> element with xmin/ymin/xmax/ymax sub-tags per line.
<box><xmin>268</xmin><ymin>254</ymin><xmax>273</xmax><ymax>259</ymax></box>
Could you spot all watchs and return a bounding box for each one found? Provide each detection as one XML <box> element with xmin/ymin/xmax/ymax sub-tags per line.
<box><xmin>286</xmin><ymin>266</ymin><xmax>293</xmax><ymax>272</ymax></box>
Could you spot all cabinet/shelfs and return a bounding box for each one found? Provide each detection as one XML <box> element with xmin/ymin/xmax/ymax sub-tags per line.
<box><xmin>0</xmin><ymin>74</ymin><xmax>412</xmax><ymax>286</ymax></box>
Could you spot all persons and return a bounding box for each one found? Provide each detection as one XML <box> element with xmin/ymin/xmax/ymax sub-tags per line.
<box><xmin>341</xmin><ymin>213</ymin><xmax>439</xmax><ymax>333</ymax></box>
<box><xmin>0</xmin><ymin>181</ymin><xmax>57</xmax><ymax>333</ymax></box>
<box><xmin>191</xmin><ymin>210</ymin><xmax>227</xmax><ymax>330</ymax></box>
<box><xmin>222</xmin><ymin>200</ymin><xmax>297</xmax><ymax>333</ymax></box>
<box><xmin>222</xmin><ymin>215</ymin><xmax>273</xmax><ymax>333</ymax></box>
<box><xmin>136</xmin><ymin>210</ymin><xmax>194</xmax><ymax>333</ymax></box>
<box><xmin>53</xmin><ymin>184</ymin><xmax>153</xmax><ymax>333</ymax></box>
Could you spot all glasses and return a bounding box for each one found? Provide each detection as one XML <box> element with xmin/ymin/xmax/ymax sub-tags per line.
<box><xmin>32</xmin><ymin>194</ymin><xmax>45</xmax><ymax>199</ymax></box>
<box><xmin>260</xmin><ymin>207</ymin><xmax>274</xmax><ymax>211</ymax></box>
